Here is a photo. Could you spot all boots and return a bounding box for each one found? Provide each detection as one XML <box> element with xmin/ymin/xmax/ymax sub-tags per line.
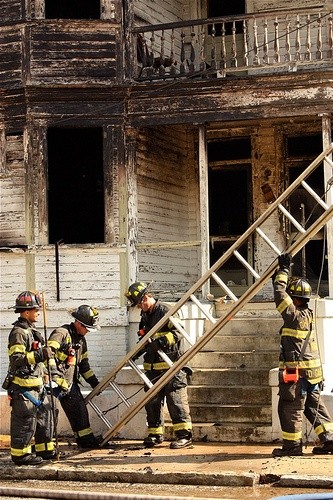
<box><xmin>312</xmin><ymin>440</ymin><xmax>333</xmax><ymax>455</ymax></box>
<box><xmin>170</xmin><ymin>438</ymin><xmax>192</xmax><ymax>449</ymax></box>
<box><xmin>49</xmin><ymin>452</ymin><xmax>65</xmax><ymax>459</ymax></box>
<box><xmin>13</xmin><ymin>453</ymin><xmax>43</xmax><ymax>465</ymax></box>
<box><xmin>272</xmin><ymin>447</ymin><xmax>304</xmax><ymax>456</ymax></box>
<box><xmin>143</xmin><ymin>435</ymin><xmax>165</xmax><ymax>446</ymax></box>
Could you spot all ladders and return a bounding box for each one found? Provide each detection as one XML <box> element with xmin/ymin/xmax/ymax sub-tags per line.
<box><xmin>76</xmin><ymin>140</ymin><xmax>333</xmax><ymax>447</ymax></box>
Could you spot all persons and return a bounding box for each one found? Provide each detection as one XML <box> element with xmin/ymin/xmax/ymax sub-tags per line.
<box><xmin>271</xmin><ymin>253</ymin><xmax>333</xmax><ymax>455</ymax></box>
<box><xmin>7</xmin><ymin>290</ymin><xmax>65</xmax><ymax>465</ymax></box>
<box><xmin>34</xmin><ymin>305</ymin><xmax>108</xmax><ymax>459</ymax></box>
<box><xmin>124</xmin><ymin>280</ymin><xmax>193</xmax><ymax>448</ymax></box>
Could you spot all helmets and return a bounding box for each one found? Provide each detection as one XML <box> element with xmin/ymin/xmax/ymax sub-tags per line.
<box><xmin>285</xmin><ymin>277</ymin><xmax>312</xmax><ymax>300</ymax></box>
<box><xmin>13</xmin><ymin>291</ymin><xmax>43</xmax><ymax>313</ymax></box>
<box><xmin>124</xmin><ymin>280</ymin><xmax>154</xmax><ymax>308</ymax></box>
<box><xmin>71</xmin><ymin>305</ymin><xmax>101</xmax><ymax>332</ymax></box>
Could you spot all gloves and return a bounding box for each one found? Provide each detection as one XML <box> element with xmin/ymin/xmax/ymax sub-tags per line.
<box><xmin>43</xmin><ymin>345</ymin><xmax>56</xmax><ymax>359</ymax></box>
<box><xmin>92</xmin><ymin>382</ymin><xmax>103</xmax><ymax>396</ymax></box>
<box><xmin>276</xmin><ymin>253</ymin><xmax>293</xmax><ymax>269</ymax></box>
<box><xmin>145</xmin><ymin>338</ymin><xmax>166</xmax><ymax>354</ymax></box>
